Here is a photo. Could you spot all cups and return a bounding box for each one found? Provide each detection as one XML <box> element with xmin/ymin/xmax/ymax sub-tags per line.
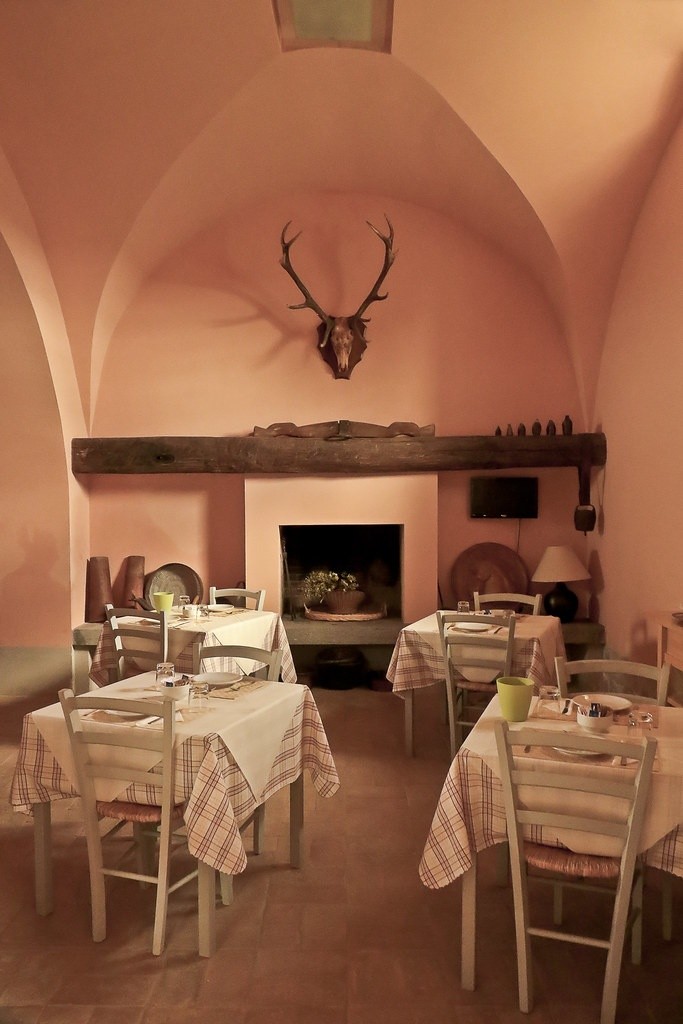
<box><xmin>153</xmin><ymin>592</ymin><xmax>174</xmax><ymax>615</ymax></box>
<box><xmin>457</xmin><ymin>601</ymin><xmax>469</xmax><ymax>615</ymax></box>
<box><xmin>188</xmin><ymin>682</ymin><xmax>209</xmax><ymax>713</ymax></box>
<box><xmin>156</xmin><ymin>662</ymin><xmax>174</xmax><ymax>689</ymax></box>
<box><xmin>627</xmin><ymin>712</ymin><xmax>653</xmax><ymax>739</ymax></box>
<box><xmin>496</xmin><ymin>676</ymin><xmax>535</xmax><ymax>722</ymax></box>
<box><xmin>538</xmin><ymin>686</ymin><xmax>561</xmax><ymax>716</ymax></box>
<box><xmin>178</xmin><ymin>596</ymin><xmax>190</xmax><ymax>612</ymax></box>
<box><xmin>196</xmin><ymin>604</ymin><xmax>209</xmax><ymax>623</ymax></box>
<box><xmin>504</xmin><ymin>610</ymin><xmax>515</xmax><ymax>620</ymax></box>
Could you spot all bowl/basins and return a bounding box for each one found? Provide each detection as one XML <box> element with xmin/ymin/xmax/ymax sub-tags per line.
<box><xmin>161</xmin><ymin>684</ymin><xmax>190</xmax><ymax>701</ymax></box>
<box><xmin>183</xmin><ymin>609</ymin><xmax>202</xmax><ymax>617</ymax></box>
<box><xmin>576</xmin><ymin>711</ymin><xmax>613</xmax><ymax>732</ymax></box>
<box><xmin>672</xmin><ymin>613</ymin><xmax>683</xmax><ymax>626</ymax></box>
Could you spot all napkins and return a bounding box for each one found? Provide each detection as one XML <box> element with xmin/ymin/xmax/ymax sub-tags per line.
<box><xmin>612</xmin><ymin>739</ymin><xmax>638</xmax><ymax>767</ymax></box>
<box><xmin>136</xmin><ymin>711</ymin><xmax>184</xmax><ymax>729</ymax></box>
<box><xmin>543</xmin><ymin>698</ymin><xmax>574</xmax><ymax>715</ymax></box>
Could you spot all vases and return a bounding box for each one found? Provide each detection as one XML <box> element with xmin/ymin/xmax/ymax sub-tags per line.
<box><xmin>323</xmin><ymin>590</ymin><xmax>365</xmax><ymax>614</ymax></box>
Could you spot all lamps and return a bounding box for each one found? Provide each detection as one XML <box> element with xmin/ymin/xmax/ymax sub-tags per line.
<box><xmin>531</xmin><ymin>545</ymin><xmax>592</xmax><ymax>622</ymax></box>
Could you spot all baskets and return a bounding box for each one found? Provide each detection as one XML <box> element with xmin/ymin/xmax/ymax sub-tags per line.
<box><xmin>324</xmin><ymin>590</ymin><xmax>364</xmax><ymax>614</ymax></box>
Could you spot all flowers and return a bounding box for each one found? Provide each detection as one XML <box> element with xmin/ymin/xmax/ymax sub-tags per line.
<box><xmin>298</xmin><ymin>569</ymin><xmax>359</xmax><ymax>604</ymax></box>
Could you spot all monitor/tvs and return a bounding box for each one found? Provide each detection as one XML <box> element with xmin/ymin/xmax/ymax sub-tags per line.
<box><xmin>470</xmin><ymin>476</ymin><xmax>538</xmax><ymax>518</ymax></box>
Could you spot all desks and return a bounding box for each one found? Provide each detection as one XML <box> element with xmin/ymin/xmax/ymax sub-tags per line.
<box><xmin>386</xmin><ymin>610</ymin><xmax>570</xmax><ymax>758</ymax></box>
<box><xmin>9</xmin><ymin>670</ymin><xmax>341</xmax><ymax>958</ymax></box>
<box><xmin>419</xmin><ymin>692</ymin><xmax>683</xmax><ymax>993</ymax></box>
<box><xmin>88</xmin><ymin>604</ymin><xmax>298</xmax><ymax>684</ymax></box>
<box><xmin>652</xmin><ymin>610</ymin><xmax>683</xmax><ymax>708</ymax></box>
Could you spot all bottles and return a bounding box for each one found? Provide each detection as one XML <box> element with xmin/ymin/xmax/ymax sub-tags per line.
<box><xmin>495</xmin><ymin>415</ymin><xmax>573</xmax><ymax>436</ymax></box>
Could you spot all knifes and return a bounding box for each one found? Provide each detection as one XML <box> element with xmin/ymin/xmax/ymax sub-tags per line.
<box><xmin>562</xmin><ymin>700</ymin><xmax>570</xmax><ymax>714</ymax></box>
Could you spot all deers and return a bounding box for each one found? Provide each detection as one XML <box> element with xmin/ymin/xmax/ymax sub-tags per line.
<box><xmin>278</xmin><ymin>212</ymin><xmax>400</xmax><ymax>374</ymax></box>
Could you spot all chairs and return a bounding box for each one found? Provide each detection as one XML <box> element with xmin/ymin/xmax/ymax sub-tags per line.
<box><xmin>57</xmin><ymin>688</ymin><xmax>234</xmax><ymax>956</ymax></box>
<box><xmin>105</xmin><ymin>603</ymin><xmax>169</xmax><ymax>681</ymax></box>
<box><xmin>210</xmin><ymin>586</ymin><xmax>266</xmax><ymax>611</ymax></box>
<box><xmin>495</xmin><ymin>719</ymin><xmax>658</xmax><ymax>1024</ymax></box>
<box><xmin>554</xmin><ymin>652</ymin><xmax>674</xmax><ymax>941</ymax></box>
<box><xmin>435</xmin><ymin>610</ymin><xmax>517</xmax><ymax>762</ymax></box>
<box><xmin>473</xmin><ymin>591</ymin><xmax>542</xmax><ymax>616</ymax></box>
<box><xmin>146</xmin><ymin>642</ymin><xmax>282</xmax><ymax>856</ymax></box>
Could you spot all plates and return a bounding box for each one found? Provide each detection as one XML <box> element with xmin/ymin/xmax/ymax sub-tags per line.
<box><xmin>195</xmin><ymin>672</ymin><xmax>243</xmax><ymax>685</ymax></box>
<box><xmin>145</xmin><ymin>563</ymin><xmax>204</xmax><ymax>609</ymax></box>
<box><xmin>144</xmin><ymin>616</ymin><xmax>181</xmax><ymax>622</ymax></box>
<box><xmin>483</xmin><ymin>610</ymin><xmax>514</xmax><ymax>615</ymax></box>
<box><xmin>104</xmin><ymin>700</ymin><xmax>163</xmax><ymax>716</ymax></box>
<box><xmin>455</xmin><ymin>622</ymin><xmax>491</xmax><ymax>631</ymax></box>
<box><xmin>573</xmin><ymin>694</ymin><xmax>633</xmax><ymax>710</ymax></box>
<box><xmin>204</xmin><ymin>604</ymin><xmax>234</xmax><ymax>611</ymax></box>
<box><xmin>552</xmin><ymin>731</ymin><xmax>608</xmax><ymax>754</ymax></box>
